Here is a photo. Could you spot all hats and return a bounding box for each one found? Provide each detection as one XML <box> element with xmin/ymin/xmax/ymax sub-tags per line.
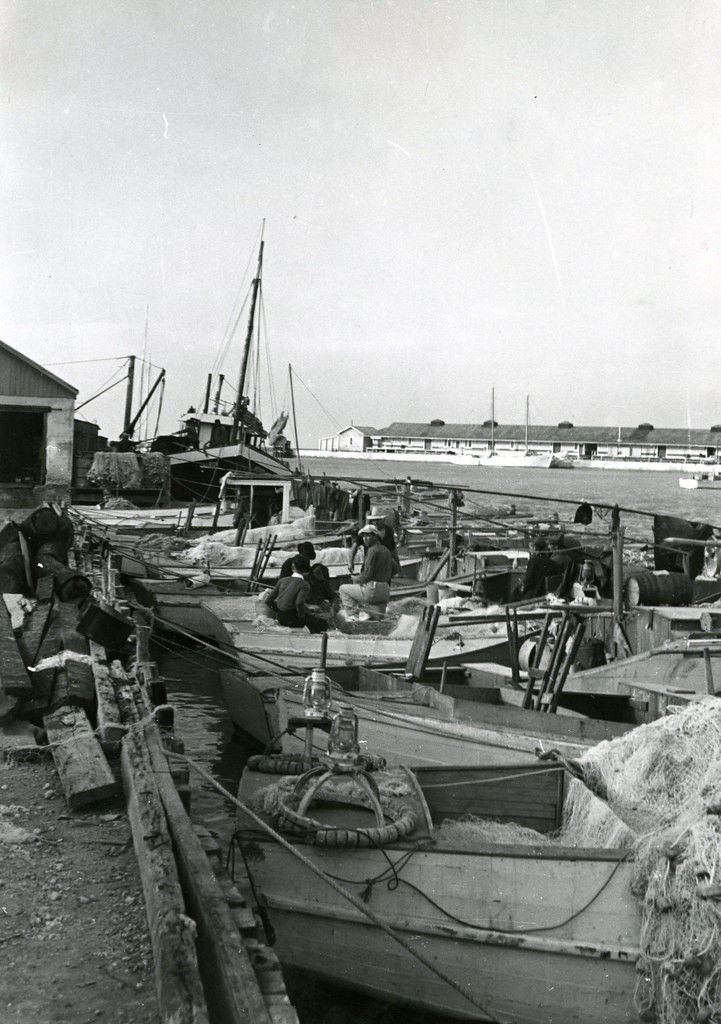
<box><xmin>293</xmin><ymin>555</ymin><xmax>309</xmax><ymax>572</ymax></box>
<box><xmin>258</xmin><ymin>588</ymin><xmax>272</xmax><ymax>600</ymax></box>
<box><xmin>310</xmin><ymin>563</ymin><xmax>329</xmax><ymax>586</ymax></box>
<box><xmin>547</xmin><ymin>533</ymin><xmax>568</xmax><ymax>549</ymax></box>
<box><xmin>298</xmin><ymin>542</ymin><xmax>317</xmax><ymax>560</ymax></box>
<box><xmin>358</xmin><ymin>525</ymin><xmax>384</xmax><ymax>540</ymax></box>
<box><xmin>366</xmin><ymin>507</ymin><xmax>388</xmax><ymax>520</ymax></box>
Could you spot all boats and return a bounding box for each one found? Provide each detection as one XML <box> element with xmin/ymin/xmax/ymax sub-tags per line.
<box><xmin>677</xmin><ymin>476</ymin><xmax>721</xmax><ymax>490</ymax></box>
<box><xmin>43</xmin><ymin>470</ymin><xmax>721</xmax><ymax>1023</ymax></box>
<box><xmin>450</xmin><ymin>387</ymin><xmax>572</xmax><ymax>469</ymax></box>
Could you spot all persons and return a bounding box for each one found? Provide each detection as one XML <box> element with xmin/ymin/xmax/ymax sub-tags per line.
<box><xmin>521</xmin><ymin>541</ymin><xmax>561</xmax><ymax>598</ymax></box>
<box><xmin>339</xmin><ymin>524</ymin><xmax>393</xmax><ymax>621</ymax></box>
<box><xmin>279</xmin><ymin>542</ymin><xmax>316</xmax><ymax>578</ymax></box>
<box><xmin>302</xmin><ymin>563</ymin><xmax>338</xmax><ymax>611</ymax></box>
<box><xmin>265</xmin><ymin>556</ymin><xmax>327</xmax><ymax>634</ymax></box>
<box><xmin>347</xmin><ymin>507</ymin><xmax>400</xmax><ymax>587</ymax></box>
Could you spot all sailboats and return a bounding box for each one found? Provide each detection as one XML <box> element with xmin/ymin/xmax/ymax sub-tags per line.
<box><xmin>109</xmin><ymin>241</ymin><xmax>295</xmax><ymax>503</ymax></box>
